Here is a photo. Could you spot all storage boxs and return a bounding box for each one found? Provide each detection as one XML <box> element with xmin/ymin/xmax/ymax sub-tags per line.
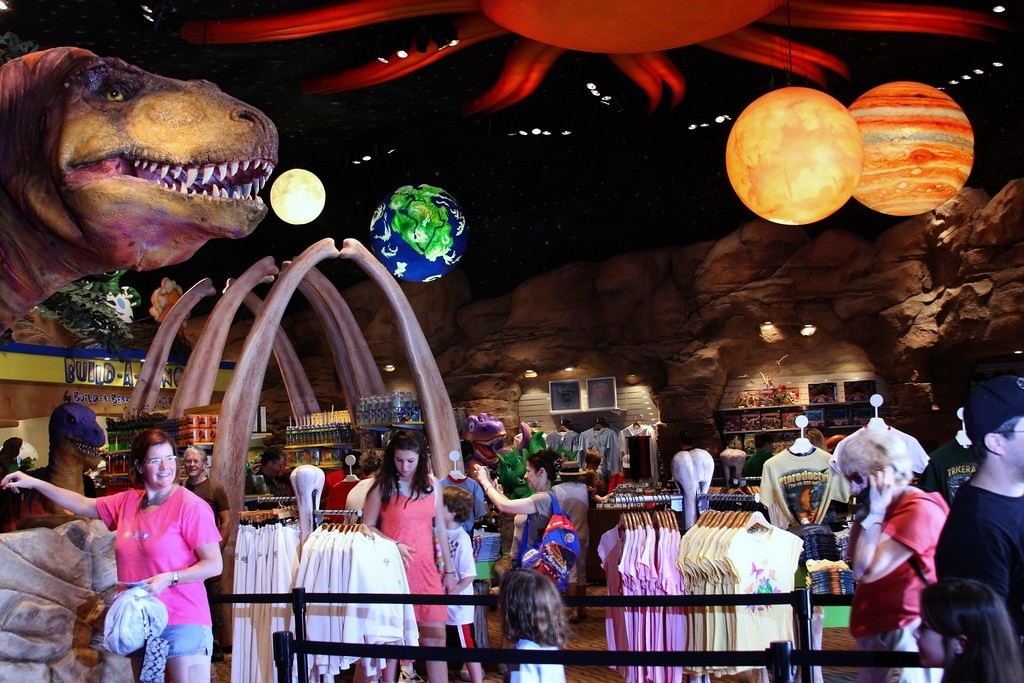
<box><xmin>720</xmin><ymin>380</ymin><xmax>875</xmax><ymax>452</ymax></box>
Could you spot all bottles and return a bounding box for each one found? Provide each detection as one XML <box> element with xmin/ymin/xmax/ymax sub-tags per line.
<box><xmin>285</xmin><ymin>423</ymin><xmax>352</xmax><ymax>445</ymax></box>
<box><xmin>107</xmin><ymin>405</ymin><xmax>153</xmax><ymax>451</ymax></box>
<box><xmin>356</xmin><ymin>391</ymin><xmax>421</xmax><ymax>424</ymax></box>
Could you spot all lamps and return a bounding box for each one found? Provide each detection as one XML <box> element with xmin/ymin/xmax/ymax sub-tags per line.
<box><xmin>848</xmin><ymin>82</ymin><xmax>975</xmax><ymax>215</ymax></box>
<box><xmin>726</xmin><ymin>1</ymin><xmax>865</xmax><ymax>225</ymax></box>
<box><xmin>270</xmin><ymin>168</ymin><xmax>325</xmax><ymax>224</ymax></box>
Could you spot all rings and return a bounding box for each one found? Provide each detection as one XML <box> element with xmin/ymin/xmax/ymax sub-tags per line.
<box><xmin>402</xmin><ymin>557</ymin><xmax>405</xmax><ymax>560</ymax></box>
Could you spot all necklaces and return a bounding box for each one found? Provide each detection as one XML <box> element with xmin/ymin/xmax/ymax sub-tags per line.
<box><xmin>146</xmin><ymin>493</ymin><xmax>168</xmax><ymax>505</ymax></box>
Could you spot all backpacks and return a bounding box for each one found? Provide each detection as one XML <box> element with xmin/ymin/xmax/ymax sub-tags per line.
<box><xmin>516</xmin><ymin>492</ymin><xmax>578</xmax><ymax>597</ymax></box>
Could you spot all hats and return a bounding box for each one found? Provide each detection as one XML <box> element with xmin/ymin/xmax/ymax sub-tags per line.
<box><xmin>963</xmin><ymin>375</ymin><xmax>1024</xmax><ymax>445</ymax></box>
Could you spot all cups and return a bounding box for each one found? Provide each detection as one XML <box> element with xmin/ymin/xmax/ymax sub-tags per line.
<box><xmin>178</xmin><ymin>414</ymin><xmax>219</xmax><ymax>443</ymax></box>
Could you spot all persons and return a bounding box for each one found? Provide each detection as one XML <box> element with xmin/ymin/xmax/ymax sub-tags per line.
<box><xmin>322</xmin><ymin>449</ymin><xmax>385</xmax><ymax>529</ymax></box>
<box><xmin>581</xmin><ymin>450</ymin><xmax>630</xmax><ymax>507</ymax></box>
<box><xmin>499</xmin><ymin>569</ymin><xmax>568</xmax><ymax>683</ymax></box>
<box><xmin>253</xmin><ymin>446</ymin><xmax>288</xmax><ymax>528</ymax></box>
<box><xmin>183</xmin><ymin>446</ymin><xmax>232</xmax><ymax>663</ymax></box>
<box><xmin>361</xmin><ymin>429</ymin><xmax>456</xmax><ymax>683</ymax></box>
<box><xmin>434</xmin><ymin>485</ymin><xmax>484</xmax><ymax>683</ymax></box>
<box><xmin>913</xmin><ymin>579</ymin><xmax>1024</xmax><ymax>683</ymax></box>
<box><xmin>0</xmin><ymin>429</ymin><xmax>223</xmax><ymax>683</ymax></box>
<box><xmin>742</xmin><ymin>432</ymin><xmax>775</xmax><ymax>486</ymax></box>
<box><xmin>839</xmin><ymin>428</ymin><xmax>950</xmax><ymax>683</ymax></box>
<box><xmin>934</xmin><ymin>376</ymin><xmax>1024</xmax><ymax>643</ymax></box>
<box><xmin>550</xmin><ymin>462</ymin><xmax>589</xmax><ymax>622</ymax></box>
<box><xmin>473</xmin><ymin>450</ymin><xmax>560</xmax><ymax>572</ymax></box>
<box><xmin>806</xmin><ymin>428</ymin><xmax>829</xmax><ymax>453</ymax></box>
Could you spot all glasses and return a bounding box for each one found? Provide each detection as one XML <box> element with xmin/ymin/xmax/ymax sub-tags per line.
<box><xmin>143</xmin><ymin>456</ymin><xmax>178</xmax><ymax>466</ymax></box>
<box><xmin>844</xmin><ymin>471</ymin><xmax>866</xmax><ymax>484</ymax></box>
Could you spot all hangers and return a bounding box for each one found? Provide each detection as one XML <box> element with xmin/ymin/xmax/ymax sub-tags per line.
<box><xmin>633</xmin><ymin>416</ymin><xmax>646</xmax><ymax>429</ymax></box>
<box><xmin>615</xmin><ymin>495</ymin><xmax>678</xmax><ymax>533</ymax></box>
<box><xmin>558</xmin><ymin>419</ymin><xmax>568</xmax><ymax>434</ymax></box>
<box><xmin>594</xmin><ymin>418</ymin><xmax>602</xmax><ymax>430</ymax></box>
<box><xmin>696</xmin><ymin>477</ymin><xmax>772</xmax><ymax>532</ymax></box>
<box><xmin>237</xmin><ymin>496</ymin><xmax>297</xmax><ymax>525</ymax></box>
<box><xmin>321</xmin><ymin>509</ymin><xmax>374</xmax><ymax>538</ymax></box>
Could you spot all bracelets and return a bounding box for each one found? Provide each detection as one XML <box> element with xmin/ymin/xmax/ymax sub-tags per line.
<box><xmin>485</xmin><ymin>486</ymin><xmax>492</xmax><ymax>492</ymax></box>
<box><xmin>873</xmin><ymin>520</ymin><xmax>883</xmax><ymax>524</ymax></box>
<box><xmin>444</xmin><ymin>572</ymin><xmax>454</xmax><ymax>574</ymax></box>
<box><xmin>395</xmin><ymin>540</ymin><xmax>400</xmax><ymax>545</ymax></box>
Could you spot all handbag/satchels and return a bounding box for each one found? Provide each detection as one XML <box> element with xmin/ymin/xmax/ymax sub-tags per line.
<box><xmin>141</xmin><ymin>607</ymin><xmax>170</xmax><ymax>683</ymax></box>
<box><xmin>102</xmin><ymin>586</ymin><xmax>168</xmax><ymax>656</ymax></box>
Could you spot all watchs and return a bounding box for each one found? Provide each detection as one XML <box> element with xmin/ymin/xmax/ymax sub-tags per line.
<box><xmin>172</xmin><ymin>571</ymin><xmax>179</xmax><ymax>585</ymax></box>
